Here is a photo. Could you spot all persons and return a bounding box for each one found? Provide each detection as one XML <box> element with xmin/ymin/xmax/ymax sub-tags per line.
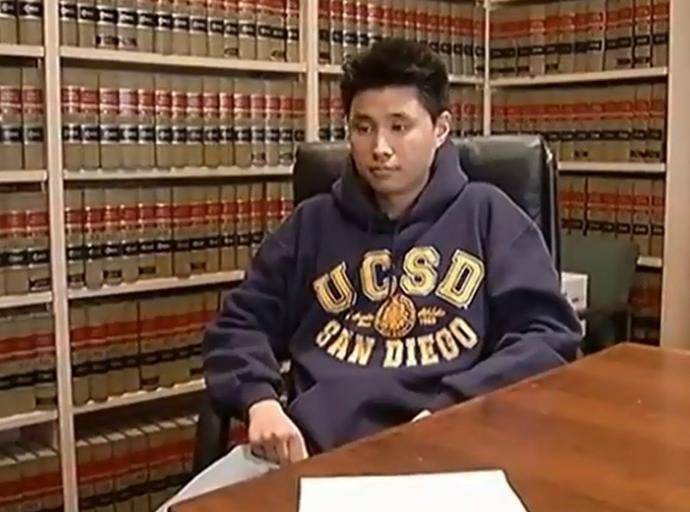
<box><xmin>158</xmin><ymin>41</ymin><xmax>583</xmax><ymax>512</ymax></box>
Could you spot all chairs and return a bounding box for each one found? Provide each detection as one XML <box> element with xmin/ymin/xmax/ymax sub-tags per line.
<box><xmin>193</xmin><ymin>135</ymin><xmax>561</xmax><ymax>473</ymax></box>
<box><xmin>558</xmin><ymin>234</ymin><xmax>641</xmax><ymax>365</ymax></box>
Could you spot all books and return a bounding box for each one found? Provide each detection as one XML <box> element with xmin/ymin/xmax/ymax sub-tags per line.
<box><xmin>316</xmin><ymin>0</ymin><xmax>485</xmax><ymax>143</ymax></box>
<box><xmin>0</xmin><ymin>0</ymin><xmax>304</xmax><ymax>512</ymax></box>
<box><xmin>490</xmin><ymin>1</ymin><xmax>669</xmax><ymax>346</ymax></box>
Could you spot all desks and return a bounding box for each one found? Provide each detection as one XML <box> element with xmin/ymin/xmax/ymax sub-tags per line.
<box><xmin>166</xmin><ymin>341</ymin><xmax>689</xmax><ymax>511</ymax></box>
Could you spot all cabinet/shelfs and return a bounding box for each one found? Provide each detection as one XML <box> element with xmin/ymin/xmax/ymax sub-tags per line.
<box><xmin>0</xmin><ymin>2</ymin><xmax>66</xmax><ymax>511</ymax></box>
<box><xmin>490</xmin><ymin>2</ymin><xmax>671</xmax><ymax>348</ymax></box>
<box><xmin>63</xmin><ymin>1</ymin><xmax>312</xmax><ymax>511</ymax></box>
<box><xmin>311</xmin><ymin>1</ymin><xmax>489</xmax><ymax>146</ymax></box>
